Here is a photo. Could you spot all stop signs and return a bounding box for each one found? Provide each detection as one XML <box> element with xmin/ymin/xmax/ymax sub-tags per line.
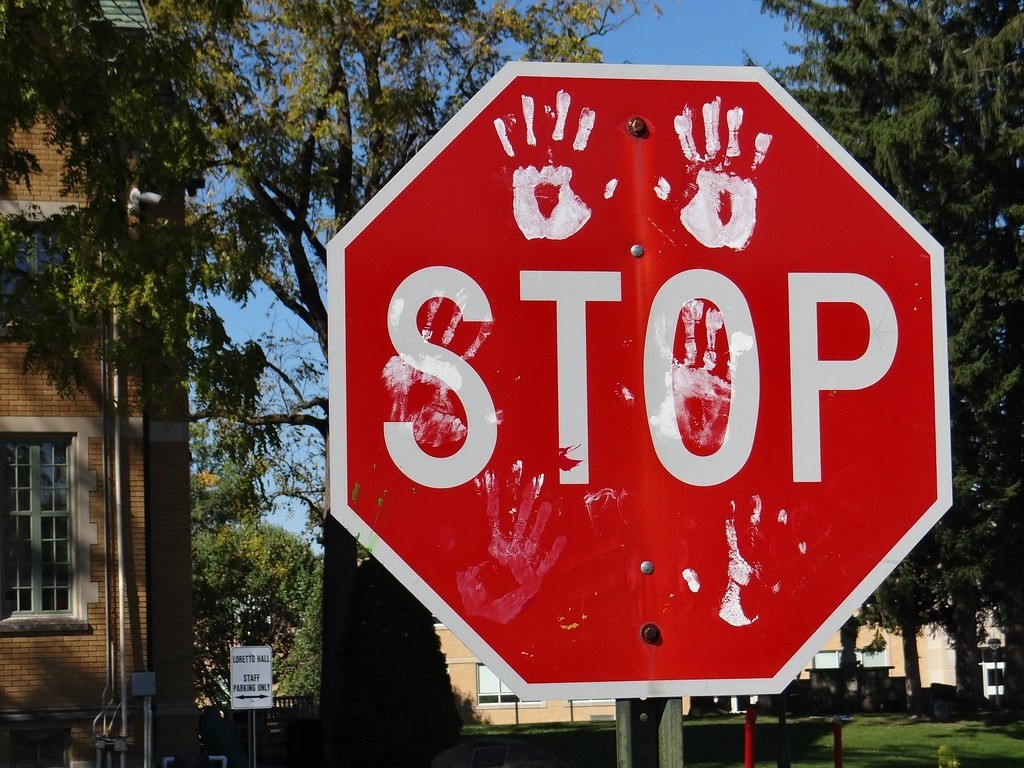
<box><xmin>327</xmin><ymin>56</ymin><xmax>955</xmax><ymax>708</ymax></box>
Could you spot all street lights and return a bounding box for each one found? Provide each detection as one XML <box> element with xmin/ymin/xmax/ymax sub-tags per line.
<box><xmin>988</xmin><ymin>639</ymin><xmax>1002</xmax><ymax>706</ymax></box>
